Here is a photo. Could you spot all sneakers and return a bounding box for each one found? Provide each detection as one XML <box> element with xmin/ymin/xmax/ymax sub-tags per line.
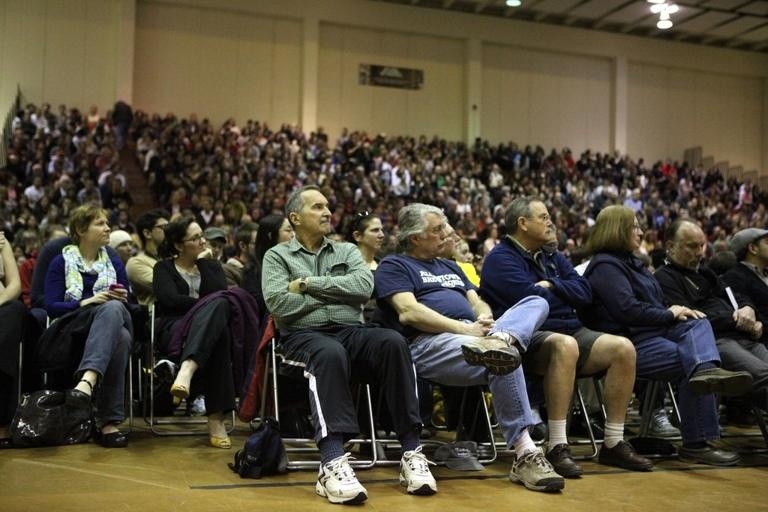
<box><xmin>677</xmin><ymin>442</ymin><xmax>743</xmax><ymax>467</ymax></box>
<box><xmin>154</xmin><ymin>358</ymin><xmax>178</xmax><ymax>385</ymax></box>
<box><xmin>188</xmin><ymin>395</ymin><xmax>208</xmax><ymax>418</ymax></box>
<box><xmin>313</xmin><ymin>455</ymin><xmax>368</xmax><ymax>506</ymax></box>
<box><xmin>510</xmin><ymin>447</ymin><xmax>566</xmax><ymax>493</ymax></box>
<box><xmin>544</xmin><ymin>443</ymin><xmax>585</xmax><ymax>477</ymax></box>
<box><xmin>460</xmin><ymin>335</ymin><xmax>522</xmax><ymax>378</ymax></box>
<box><xmin>638</xmin><ymin>408</ymin><xmax>682</xmax><ymax>442</ymax></box>
<box><xmin>687</xmin><ymin>366</ymin><xmax>753</xmax><ymax>398</ymax></box>
<box><xmin>399</xmin><ymin>447</ymin><xmax>440</xmax><ymax>496</ymax></box>
<box><xmin>599</xmin><ymin>440</ymin><xmax>653</xmax><ymax>472</ymax></box>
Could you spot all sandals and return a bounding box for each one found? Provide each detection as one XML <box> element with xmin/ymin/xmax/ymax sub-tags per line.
<box><xmin>205</xmin><ymin>418</ymin><xmax>231</xmax><ymax>450</ymax></box>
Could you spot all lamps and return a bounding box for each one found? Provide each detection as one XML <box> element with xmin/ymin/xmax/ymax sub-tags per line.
<box><xmin>648</xmin><ymin>0</ymin><xmax>680</xmax><ymax>30</ymax></box>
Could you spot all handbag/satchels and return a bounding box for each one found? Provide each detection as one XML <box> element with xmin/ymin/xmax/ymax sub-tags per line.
<box><xmin>8</xmin><ymin>388</ymin><xmax>94</xmax><ymax>448</ymax></box>
<box><xmin>232</xmin><ymin>417</ymin><xmax>281</xmax><ymax>480</ymax></box>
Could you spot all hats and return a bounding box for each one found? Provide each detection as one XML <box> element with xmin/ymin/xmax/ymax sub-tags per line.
<box><xmin>204</xmin><ymin>225</ymin><xmax>229</xmax><ymax>245</ymax></box>
<box><xmin>730</xmin><ymin>227</ymin><xmax>768</xmax><ymax>254</ymax></box>
<box><xmin>434</xmin><ymin>439</ymin><xmax>486</xmax><ymax>472</ymax></box>
<box><xmin>107</xmin><ymin>229</ymin><xmax>133</xmax><ymax>251</ymax></box>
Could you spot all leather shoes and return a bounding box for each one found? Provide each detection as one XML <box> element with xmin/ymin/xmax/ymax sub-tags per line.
<box><xmin>65</xmin><ymin>377</ymin><xmax>94</xmax><ymax>411</ymax></box>
<box><xmin>94</xmin><ymin>419</ymin><xmax>130</xmax><ymax>448</ymax></box>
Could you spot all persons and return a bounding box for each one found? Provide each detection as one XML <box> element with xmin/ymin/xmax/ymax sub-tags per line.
<box><xmin>0</xmin><ymin>95</ymin><xmax>768</xmax><ymax>507</ymax></box>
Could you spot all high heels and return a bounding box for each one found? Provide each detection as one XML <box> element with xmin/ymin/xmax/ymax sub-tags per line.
<box><xmin>168</xmin><ymin>382</ymin><xmax>191</xmax><ymax>407</ymax></box>
